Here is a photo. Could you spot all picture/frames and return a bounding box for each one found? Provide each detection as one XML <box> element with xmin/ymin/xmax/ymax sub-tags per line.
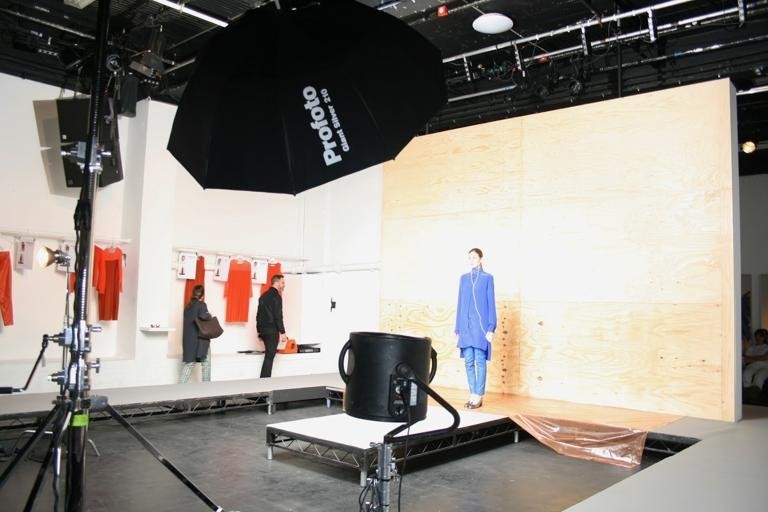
<box><xmin>757</xmin><ymin>274</ymin><xmax>768</xmax><ymax>335</ymax></box>
<box><xmin>741</xmin><ymin>273</ymin><xmax>753</xmax><ymax>339</ymax></box>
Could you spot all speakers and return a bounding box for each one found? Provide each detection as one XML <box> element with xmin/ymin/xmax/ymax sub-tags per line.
<box><xmin>57</xmin><ymin>96</ymin><xmax>123</xmax><ymax>187</ymax></box>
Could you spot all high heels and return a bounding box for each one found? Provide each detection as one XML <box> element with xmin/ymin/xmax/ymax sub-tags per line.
<box><xmin>463</xmin><ymin>400</ymin><xmax>474</xmax><ymax>409</ymax></box>
<box><xmin>469</xmin><ymin>396</ymin><xmax>483</xmax><ymax>410</ymax></box>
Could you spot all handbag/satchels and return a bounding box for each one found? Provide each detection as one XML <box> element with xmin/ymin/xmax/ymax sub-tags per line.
<box><xmin>194</xmin><ymin>301</ymin><xmax>224</xmax><ymax>341</ymax></box>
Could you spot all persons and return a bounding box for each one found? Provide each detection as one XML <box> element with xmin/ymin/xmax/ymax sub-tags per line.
<box><xmin>454</xmin><ymin>248</ymin><xmax>496</xmax><ymax>410</ymax></box>
<box><xmin>178</xmin><ymin>285</ymin><xmax>213</xmax><ymax>383</ymax></box>
<box><xmin>258</xmin><ymin>275</ymin><xmax>288</xmax><ymax>379</ymax></box>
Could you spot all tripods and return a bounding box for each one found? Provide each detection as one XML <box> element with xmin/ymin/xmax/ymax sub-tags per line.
<box><xmin>0</xmin><ymin>188</ymin><xmax>223</xmax><ymax>512</ymax></box>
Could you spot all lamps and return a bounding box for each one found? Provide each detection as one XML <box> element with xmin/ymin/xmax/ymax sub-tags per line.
<box><xmin>37</xmin><ymin>242</ymin><xmax>101</xmax><ymax>463</ymax></box>
<box><xmin>470</xmin><ymin>11</ymin><xmax>516</xmax><ymax>35</ymax></box>
